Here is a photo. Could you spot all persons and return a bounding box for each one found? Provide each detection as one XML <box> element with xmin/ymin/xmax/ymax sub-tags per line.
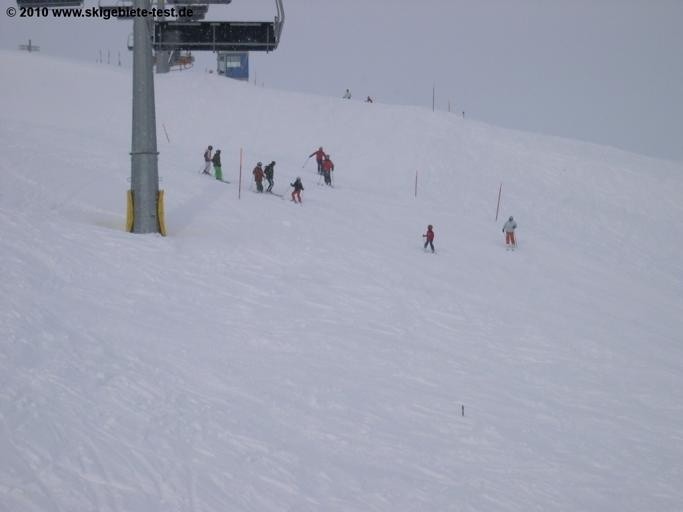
<box><xmin>290</xmin><ymin>176</ymin><xmax>304</xmax><ymax>203</ymax></box>
<box><xmin>204</xmin><ymin>146</ymin><xmax>223</xmax><ymax>180</ymax></box>
<box><xmin>253</xmin><ymin>161</ymin><xmax>276</xmax><ymax>193</ymax></box>
<box><xmin>309</xmin><ymin>147</ymin><xmax>334</xmax><ymax>186</ymax></box>
<box><xmin>423</xmin><ymin>225</ymin><xmax>434</xmax><ymax>253</ymax></box>
<box><xmin>343</xmin><ymin>89</ymin><xmax>373</xmax><ymax>103</ymax></box>
<box><xmin>502</xmin><ymin>216</ymin><xmax>517</xmax><ymax>244</ymax></box>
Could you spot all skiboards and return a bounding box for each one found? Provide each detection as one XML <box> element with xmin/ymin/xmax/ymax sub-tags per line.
<box><xmin>290</xmin><ymin>196</ymin><xmax>304</xmax><ymax>206</ymax></box>
<box><xmin>212</xmin><ymin>175</ymin><xmax>230</xmax><ymax>184</ymax></box>
<box><xmin>506</xmin><ymin>244</ymin><xmax>514</xmax><ymax>255</ymax></box>
<box><xmin>263</xmin><ymin>188</ymin><xmax>285</xmax><ymax>198</ymax></box>
